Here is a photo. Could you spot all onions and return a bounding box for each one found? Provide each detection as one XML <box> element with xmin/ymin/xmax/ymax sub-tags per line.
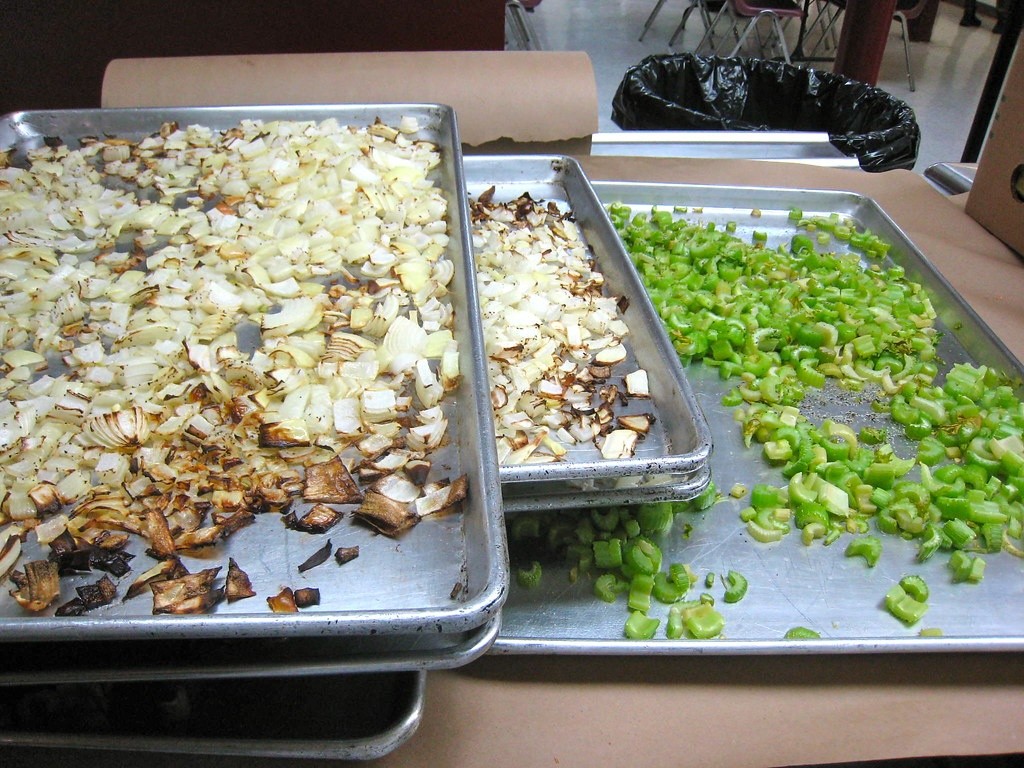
<box><xmin>0</xmin><ymin>114</ymin><xmax>657</xmax><ymax>618</ymax></box>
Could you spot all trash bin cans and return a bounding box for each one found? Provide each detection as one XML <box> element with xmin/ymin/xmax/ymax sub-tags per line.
<box><xmin>610</xmin><ymin>52</ymin><xmax>921</xmax><ymax>173</ymax></box>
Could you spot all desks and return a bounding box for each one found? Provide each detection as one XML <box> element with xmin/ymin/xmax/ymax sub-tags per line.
<box><xmin>380</xmin><ymin>156</ymin><xmax>1024</xmax><ymax>768</ymax></box>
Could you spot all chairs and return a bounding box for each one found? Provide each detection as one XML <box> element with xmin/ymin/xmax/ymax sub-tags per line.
<box><xmin>505</xmin><ymin>0</ymin><xmax>543</xmax><ymax>51</ymax></box>
<box><xmin>638</xmin><ymin>0</ymin><xmax>928</xmax><ymax>92</ymax></box>
